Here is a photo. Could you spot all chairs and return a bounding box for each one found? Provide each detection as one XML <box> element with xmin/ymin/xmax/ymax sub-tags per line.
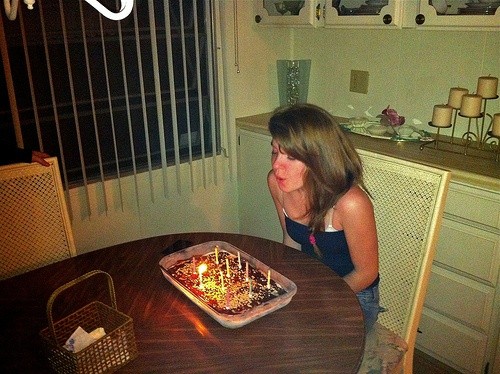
<box><xmin>0</xmin><ymin>157</ymin><xmax>77</xmax><ymax>281</ymax></box>
<box><xmin>351</xmin><ymin>149</ymin><xmax>451</xmax><ymax>374</ymax></box>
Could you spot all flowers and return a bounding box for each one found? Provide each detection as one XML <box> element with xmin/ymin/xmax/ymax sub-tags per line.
<box><xmin>376</xmin><ymin>104</ymin><xmax>406</xmax><ymax>135</ymax></box>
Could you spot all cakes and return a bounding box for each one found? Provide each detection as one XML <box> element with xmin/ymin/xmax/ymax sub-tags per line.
<box><xmin>166</xmin><ymin>250</ymin><xmax>287</xmax><ymax>315</ymax></box>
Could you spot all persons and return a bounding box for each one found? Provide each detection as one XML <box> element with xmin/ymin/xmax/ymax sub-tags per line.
<box><xmin>267</xmin><ymin>104</ymin><xmax>381</xmax><ymax>333</ymax></box>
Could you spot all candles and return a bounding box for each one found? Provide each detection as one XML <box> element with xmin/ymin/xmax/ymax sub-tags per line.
<box><xmin>448</xmin><ymin>85</ymin><xmax>469</xmax><ymax>108</ymax></box>
<box><xmin>477</xmin><ymin>74</ymin><xmax>498</xmax><ymax>98</ymax></box>
<box><xmin>492</xmin><ymin>113</ymin><xmax>500</xmax><ymax>136</ymax></box>
<box><xmin>432</xmin><ymin>104</ymin><xmax>452</xmax><ymax>126</ymax></box>
<box><xmin>193</xmin><ymin>246</ymin><xmax>271</xmax><ymax>310</ymax></box>
<box><xmin>461</xmin><ymin>91</ymin><xmax>482</xmax><ymax>116</ymax></box>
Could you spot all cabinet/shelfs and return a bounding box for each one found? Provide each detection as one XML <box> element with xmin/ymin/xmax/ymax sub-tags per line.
<box><xmin>319</xmin><ymin>0</ymin><xmax>500</xmax><ymax>31</ymax></box>
<box><xmin>246</xmin><ymin>0</ymin><xmax>319</xmax><ymax>28</ymax></box>
<box><xmin>236</xmin><ymin>128</ymin><xmax>284</xmax><ymax>242</ymax></box>
<box><xmin>412</xmin><ymin>181</ymin><xmax>500</xmax><ymax>374</ymax></box>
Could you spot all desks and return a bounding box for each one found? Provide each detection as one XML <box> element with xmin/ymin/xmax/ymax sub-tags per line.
<box><xmin>1</xmin><ymin>232</ymin><xmax>365</xmax><ymax>374</ymax></box>
